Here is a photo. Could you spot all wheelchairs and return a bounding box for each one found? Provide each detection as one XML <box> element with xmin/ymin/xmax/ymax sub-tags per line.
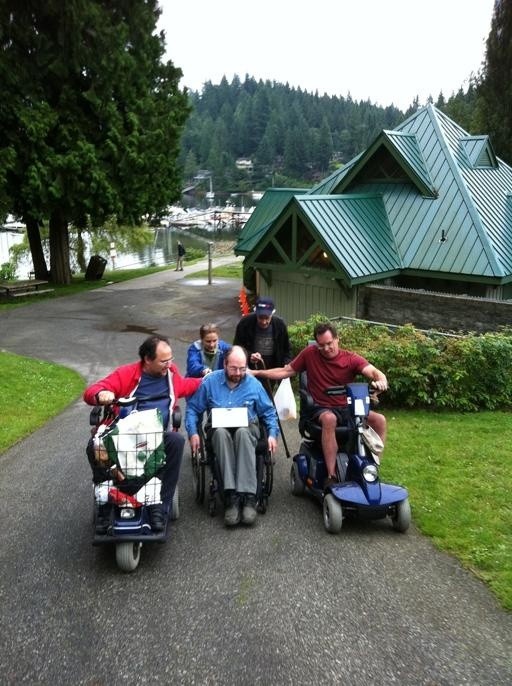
<box><xmin>191</xmin><ymin>370</ymin><xmax>277</xmax><ymax>517</ymax></box>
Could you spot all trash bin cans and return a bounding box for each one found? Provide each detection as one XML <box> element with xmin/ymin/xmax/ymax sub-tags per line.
<box><xmin>85</xmin><ymin>255</ymin><xmax>107</xmax><ymax>281</ymax></box>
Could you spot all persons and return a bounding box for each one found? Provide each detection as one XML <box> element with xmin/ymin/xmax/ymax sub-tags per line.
<box><xmin>186</xmin><ymin>345</ymin><xmax>280</xmax><ymax>528</ymax></box>
<box><xmin>186</xmin><ymin>323</ymin><xmax>231</xmax><ymax>378</ymax></box>
<box><xmin>173</xmin><ymin>239</ymin><xmax>187</xmax><ymax>272</ymax></box>
<box><xmin>84</xmin><ymin>335</ymin><xmax>207</xmax><ymax>535</ymax></box>
<box><xmin>246</xmin><ymin>323</ymin><xmax>388</xmax><ymax>494</ymax></box>
<box><xmin>233</xmin><ymin>296</ymin><xmax>288</xmax><ymax>402</ymax></box>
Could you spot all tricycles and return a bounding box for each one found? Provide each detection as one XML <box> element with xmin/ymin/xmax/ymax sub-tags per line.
<box><xmin>90</xmin><ymin>394</ymin><xmax>182</xmax><ymax>571</ymax></box>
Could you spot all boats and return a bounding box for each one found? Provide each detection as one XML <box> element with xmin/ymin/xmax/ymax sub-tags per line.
<box><xmin>3</xmin><ymin>221</ymin><xmax>27</xmax><ymax>233</ymax></box>
<box><xmin>140</xmin><ymin>206</ymin><xmax>266</xmax><ymax>233</ymax></box>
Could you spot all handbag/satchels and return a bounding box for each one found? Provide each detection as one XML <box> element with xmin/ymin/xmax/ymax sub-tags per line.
<box><xmin>102</xmin><ymin>408</ymin><xmax>166</xmax><ymax>481</ymax></box>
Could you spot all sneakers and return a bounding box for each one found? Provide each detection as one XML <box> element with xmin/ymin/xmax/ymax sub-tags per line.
<box><xmin>149</xmin><ymin>506</ymin><xmax>165</xmax><ymax>532</ymax></box>
<box><xmin>93</xmin><ymin>502</ymin><xmax>113</xmax><ymax>533</ymax></box>
<box><xmin>226</xmin><ymin>496</ymin><xmax>242</xmax><ymax>525</ymax></box>
<box><xmin>242</xmin><ymin>498</ymin><xmax>257</xmax><ymax>525</ymax></box>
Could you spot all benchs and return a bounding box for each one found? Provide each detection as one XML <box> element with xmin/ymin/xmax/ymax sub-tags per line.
<box><xmin>0</xmin><ymin>278</ymin><xmax>56</xmax><ymax>299</ymax></box>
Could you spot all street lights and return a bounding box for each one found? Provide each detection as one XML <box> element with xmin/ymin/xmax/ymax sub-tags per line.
<box><xmin>110</xmin><ymin>241</ymin><xmax>117</xmax><ymax>271</ymax></box>
<box><xmin>207</xmin><ymin>241</ymin><xmax>214</xmax><ymax>284</ymax></box>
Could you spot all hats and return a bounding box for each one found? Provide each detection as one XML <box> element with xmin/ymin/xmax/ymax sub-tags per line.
<box><xmin>253</xmin><ymin>297</ymin><xmax>275</xmax><ymax>317</ymax></box>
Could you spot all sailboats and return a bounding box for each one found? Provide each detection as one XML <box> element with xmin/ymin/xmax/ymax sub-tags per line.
<box><xmin>206</xmin><ymin>175</ymin><xmax>215</xmax><ymax>198</ymax></box>
